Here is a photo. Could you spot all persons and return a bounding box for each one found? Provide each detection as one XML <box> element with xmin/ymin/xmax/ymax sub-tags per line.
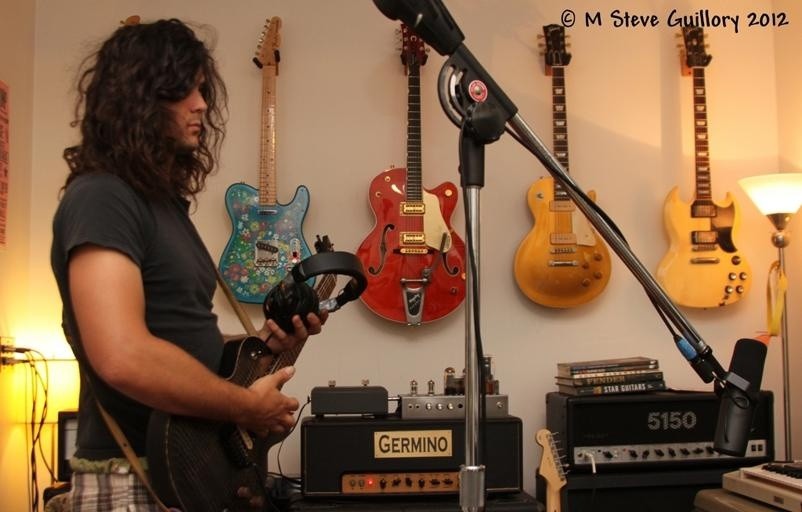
<box><xmin>49</xmin><ymin>17</ymin><xmax>325</xmax><ymax>512</ymax></box>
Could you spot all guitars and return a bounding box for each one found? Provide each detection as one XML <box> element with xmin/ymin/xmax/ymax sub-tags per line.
<box><xmin>353</xmin><ymin>24</ymin><xmax>467</xmax><ymax>326</ymax></box>
<box><xmin>535</xmin><ymin>430</ymin><xmax>570</xmax><ymax>512</ymax></box>
<box><xmin>218</xmin><ymin>16</ymin><xmax>316</xmax><ymax>304</ymax></box>
<box><xmin>656</xmin><ymin>26</ymin><xmax>752</xmax><ymax>308</ymax></box>
<box><xmin>145</xmin><ymin>234</ymin><xmax>337</xmax><ymax>512</ymax></box>
<box><xmin>514</xmin><ymin>24</ymin><xmax>611</xmax><ymax>310</ymax></box>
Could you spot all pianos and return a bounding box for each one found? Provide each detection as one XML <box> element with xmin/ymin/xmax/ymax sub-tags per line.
<box><xmin>723</xmin><ymin>462</ymin><xmax>799</xmax><ymax>510</ymax></box>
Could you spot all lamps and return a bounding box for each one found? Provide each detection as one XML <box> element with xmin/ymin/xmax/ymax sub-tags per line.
<box><xmin>740</xmin><ymin>173</ymin><xmax>802</xmax><ymax>467</ymax></box>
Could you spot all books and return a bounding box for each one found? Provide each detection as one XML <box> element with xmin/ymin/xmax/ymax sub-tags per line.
<box><xmin>555</xmin><ymin>356</ymin><xmax>666</xmax><ymax>397</ymax></box>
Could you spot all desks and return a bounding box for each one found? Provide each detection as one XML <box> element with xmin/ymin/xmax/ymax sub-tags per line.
<box><xmin>276</xmin><ymin>469</ymin><xmax>547</xmax><ymax>512</ymax></box>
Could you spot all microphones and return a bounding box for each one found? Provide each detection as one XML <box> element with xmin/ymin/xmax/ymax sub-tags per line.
<box><xmin>712</xmin><ymin>338</ymin><xmax>767</xmax><ymax>458</ymax></box>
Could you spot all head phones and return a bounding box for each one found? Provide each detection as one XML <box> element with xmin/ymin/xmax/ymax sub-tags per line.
<box><xmin>262</xmin><ymin>252</ymin><xmax>368</xmax><ymax>335</ymax></box>
